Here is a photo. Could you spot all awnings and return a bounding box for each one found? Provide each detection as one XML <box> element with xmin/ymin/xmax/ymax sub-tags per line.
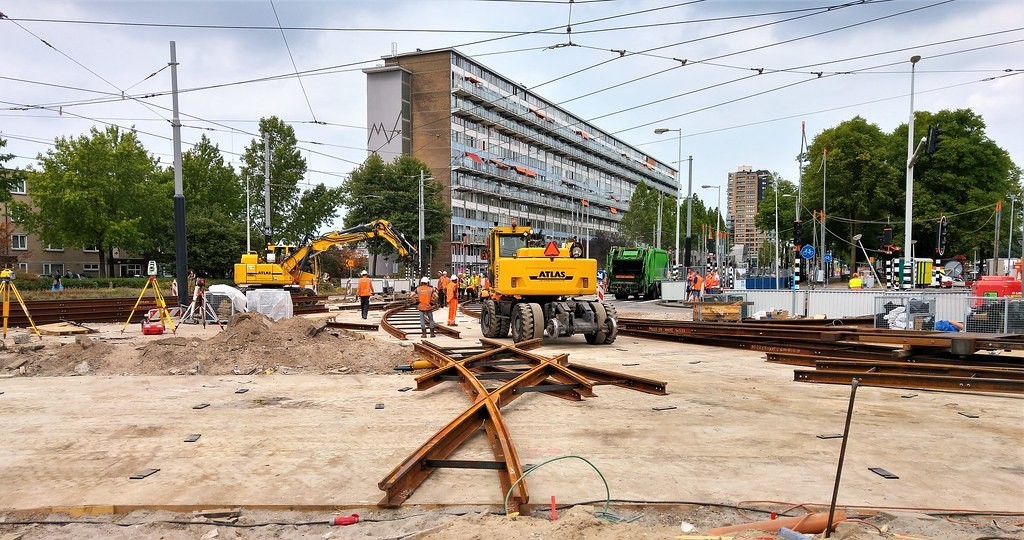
<box><xmin>464</xmin><ymin>77</ymin><xmax>651</xmax><ymax>214</ymax></box>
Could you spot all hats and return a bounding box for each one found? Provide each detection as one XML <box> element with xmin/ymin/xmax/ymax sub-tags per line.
<box><xmin>450</xmin><ymin>274</ymin><xmax>457</xmax><ymax>280</ymax></box>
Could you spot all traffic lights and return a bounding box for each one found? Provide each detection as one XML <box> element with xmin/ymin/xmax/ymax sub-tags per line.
<box><xmin>927</xmin><ymin>125</ymin><xmax>945</xmax><ymax>155</ymax></box>
<box><xmin>794</xmin><ymin>221</ymin><xmax>803</xmax><ymax>247</ymax></box>
<box><xmin>877</xmin><ymin>236</ymin><xmax>884</xmax><ymax>251</ymax></box>
<box><xmin>935</xmin><ymin>216</ymin><xmax>950</xmax><ymax>256</ymax></box>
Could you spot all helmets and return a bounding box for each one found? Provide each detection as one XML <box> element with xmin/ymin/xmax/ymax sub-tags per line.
<box><xmin>361</xmin><ymin>270</ymin><xmax>368</xmax><ymax>276</ymax></box>
<box><xmin>442</xmin><ymin>271</ymin><xmax>447</xmax><ymax>275</ymax></box>
<box><xmin>438</xmin><ymin>271</ymin><xmax>442</xmax><ymax>274</ymax></box>
<box><xmin>421</xmin><ymin>276</ymin><xmax>430</xmax><ymax>283</ymax></box>
<box><xmin>853</xmin><ymin>273</ymin><xmax>859</xmax><ymax>277</ymax></box>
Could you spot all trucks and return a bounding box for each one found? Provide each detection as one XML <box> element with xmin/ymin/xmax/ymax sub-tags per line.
<box><xmin>606</xmin><ymin>245</ymin><xmax>668</xmax><ymax>300</ymax></box>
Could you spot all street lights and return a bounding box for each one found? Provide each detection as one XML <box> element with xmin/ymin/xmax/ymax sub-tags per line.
<box><xmin>902</xmin><ymin>54</ymin><xmax>924</xmax><ymax>291</ymax></box>
<box><xmin>853</xmin><ymin>234</ymin><xmax>884</xmax><ymax>290</ymax></box>
<box><xmin>1004</xmin><ymin>192</ymin><xmax>1022</xmax><ymax>278</ymax></box>
<box><xmin>702</xmin><ymin>185</ymin><xmax>722</xmax><ymax>271</ymax></box>
<box><xmin>654</xmin><ymin>128</ymin><xmax>682</xmax><ymax>272</ymax></box>
<box><xmin>246</xmin><ymin>170</ymin><xmax>264</xmax><ymax>254</ymax></box>
<box><xmin>911</xmin><ymin>240</ymin><xmax>918</xmax><ymax>290</ymax></box>
<box><xmin>782</xmin><ymin>195</ymin><xmax>800</xmax><ymax>221</ymax></box>
<box><xmin>418</xmin><ymin>170</ymin><xmax>435</xmax><ymax>282</ymax></box>
<box><xmin>672</xmin><ymin>155</ymin><xmax>693</xmax><ymax>272</ymax></box>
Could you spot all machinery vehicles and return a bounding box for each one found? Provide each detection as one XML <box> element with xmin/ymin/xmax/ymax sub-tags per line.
<box><xmin>232</xmin><ymin>219</ymin><xmax>419</xmax><ymax>307</ymax></box>
<box><xmin>480</xmin><ymin>221</ymin><xmax>619</xmax><ymax>347</ymax></box>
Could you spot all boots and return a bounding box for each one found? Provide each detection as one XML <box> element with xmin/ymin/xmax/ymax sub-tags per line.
<box><xmin>430</xmin><ymin>328</ymin><xmax>436</xmax><ymax>337</ymax></box>
<box><xmin>421</xmin><ymin>329</ymin><xmax>427</xmax><ymax>338</ymax></box>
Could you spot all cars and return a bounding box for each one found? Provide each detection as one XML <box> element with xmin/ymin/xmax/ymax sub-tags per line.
<box><xmin>63</xmin><ymin>273</ymin><xmax>93</xmax><ymax>280</ymax></box>
<box><xmin>37</xmin><ymin>274</ymin><xmax>52</xmax><ymax>278</ymax></box>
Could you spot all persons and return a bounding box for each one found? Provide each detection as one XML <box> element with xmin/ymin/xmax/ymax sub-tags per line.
<box><xmin>188</xmin><ymin>269</ymin><xmax>196</xmax><ymax>282</ymax></box>
<box><xmin>847</xmin><ymin>273</ymin><xmax>864</xmax><ymax>291</ymax></box>
<box><xmin>684</xmin><ymin>268</ymin><xmax>719</xmax><ymax>302</ymax></box>
<box><xmin>54</xmin><ymin>269</ymin><xmax>73</xmax><ymax>284</ymax></box>
<box><xmin>357</xmin><ymin>269</ymin><xmax>479</xmax><ymax>339</ymax></box>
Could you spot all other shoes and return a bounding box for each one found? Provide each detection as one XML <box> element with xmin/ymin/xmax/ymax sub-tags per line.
<box><xmin>448</xmin><ymin>324</ymin><xmax>458</xmax><ymax>326</ymax></box>
